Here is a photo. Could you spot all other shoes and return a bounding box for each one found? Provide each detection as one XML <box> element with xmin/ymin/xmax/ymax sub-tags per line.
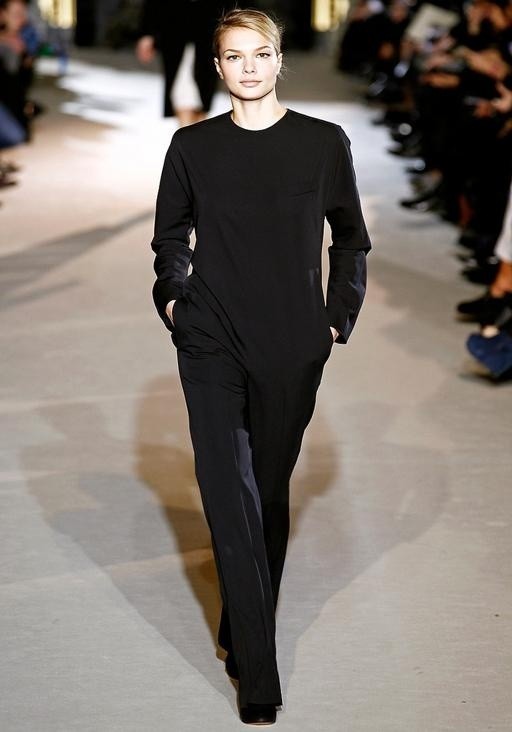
<box><xmin>457</xmin><ymin>286</ymin><xmax>511</xmax><ymax>324</ymax></box>
<box><xmin>466</xmin><ymin>333</ymin><xmax>511</xmax><ymax>381</ymax></box>
<box><xmin>368</xmin><ymin>72</ymin><xmax>504</xmax><ymax>286</ymax></box>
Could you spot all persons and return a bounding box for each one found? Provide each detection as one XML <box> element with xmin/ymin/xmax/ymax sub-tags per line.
<box><xmin>0</xmin><ymin>0</ymin><xmax>77</xmax><ymax>211</ymax></box>
<box><xmin>133</xmin><ymin>1</ymin><xmax>225</xmax><ymax>129</ymax></box>
<box><xmin>336</xmin><ymin>0</ymin><xmax>512</xmax><ymax>383</ymax></box>
<box><xmin>151</xmin><ymin>9</ymin><xmax>372</xmax><ymax>724</ymax></box>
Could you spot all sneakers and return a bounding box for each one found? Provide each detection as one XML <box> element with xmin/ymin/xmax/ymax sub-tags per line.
<box><xmin>223</xmin><ymin>643</ymin><xmax>277</xmax><ymax>725</ymax></box>
